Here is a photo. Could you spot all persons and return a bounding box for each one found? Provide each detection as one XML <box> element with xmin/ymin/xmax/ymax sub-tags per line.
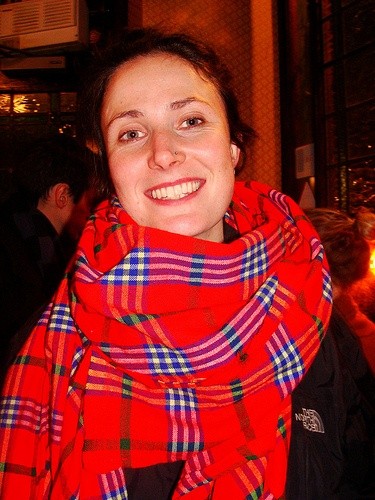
<box><xmin>301</xmin><ymin>206</ymin><xmax>375</xmax><ymax>373</ymax></box>
<box><xmin>0</xmin><ymin>131</ymin><xmax>106</xmax><ymax>388</ymax></box>
<box><xmin>0</xmin><ymin>30</ymin><xmax>375</xmax><ymax>499</ymax></box>
